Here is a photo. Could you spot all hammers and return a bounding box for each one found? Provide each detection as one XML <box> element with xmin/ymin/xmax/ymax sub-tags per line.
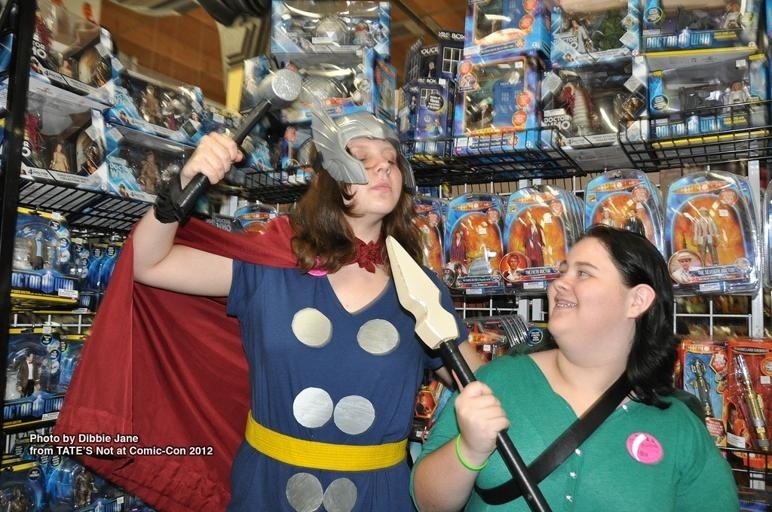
<box><xmin>174</xmin><ymin>68</ymin><xmax>303</xmax><ymax>220</ymax></box>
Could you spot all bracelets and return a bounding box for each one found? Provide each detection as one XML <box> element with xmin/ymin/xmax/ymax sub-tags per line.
<box><xmin>452</xmin><ymin>434</ymin><xmax>488</xmax><ymax>472</ymax></box>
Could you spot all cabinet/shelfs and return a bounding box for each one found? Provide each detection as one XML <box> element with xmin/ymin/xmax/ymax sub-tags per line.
<box><xmin>0</xmin><ymin>0</ymin><xmax>772</xmax><ymax>511</ymax></box>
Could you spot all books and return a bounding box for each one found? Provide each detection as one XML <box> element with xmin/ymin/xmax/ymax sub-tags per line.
<box><xmin>656</xmin><ymin>307</ymin><xmax>771</xmax><ymax>512</ymax></box>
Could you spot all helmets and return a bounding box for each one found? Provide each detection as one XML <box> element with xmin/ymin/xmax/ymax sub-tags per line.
<box><xmin>309</xmin><ymin>99</ymin><xmax>417</xmax><ymax>196</ymax></box>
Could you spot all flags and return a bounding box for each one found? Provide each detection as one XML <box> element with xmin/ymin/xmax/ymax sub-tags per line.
<box><xmin>410</xmin><ymin>312</ymin><xmax>561</xmax><ymax>440</ymax></box>
<box><xmin>0</xmin><ymin>2</ymin><xmax>267</xmax><ymax>512</ymax></box>
<box><xmin>384</xmin><ymin>231</ymin><xmax>554</xmax><ymax>512</ymax></box>
<box><xmin>269</xmin><ymin>4</ymin><xmax>772</xmax><ymax>310</ymax></box>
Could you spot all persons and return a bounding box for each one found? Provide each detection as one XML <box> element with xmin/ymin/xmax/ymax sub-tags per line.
<box><xmin>404</xmin><ymin>221</ymin><xmax>738</xmax><ymax>512</ymax></box>
<box><xmin>132</xmin><ymin>107</ymin><xmax>486</xmax><ymax>511</ymax></box>
